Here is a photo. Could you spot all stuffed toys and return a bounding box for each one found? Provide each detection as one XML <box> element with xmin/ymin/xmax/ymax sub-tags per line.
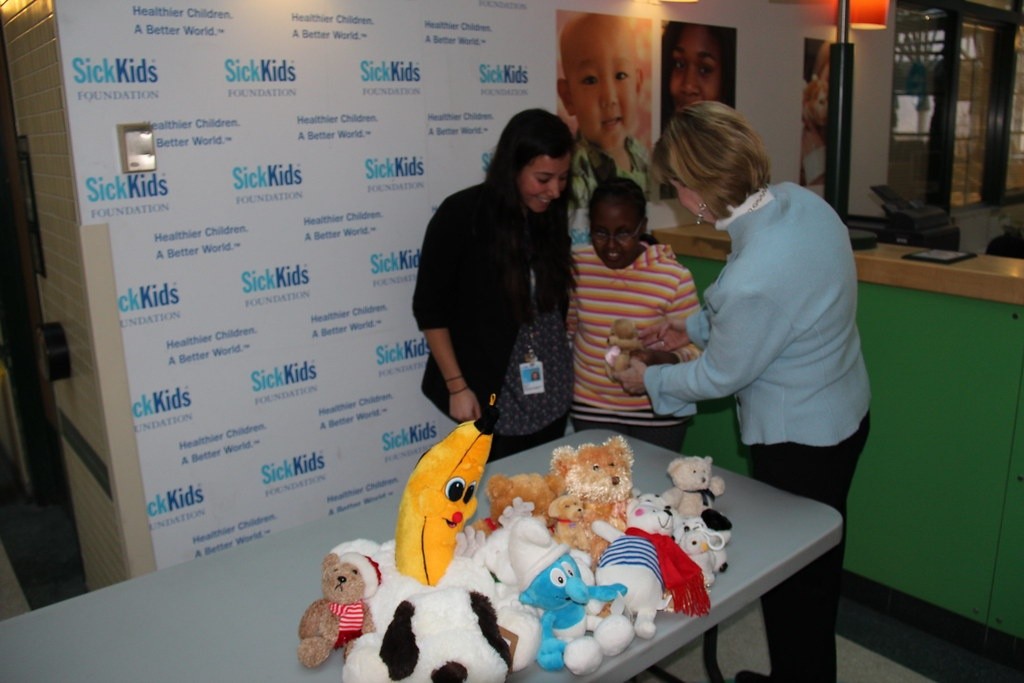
<box><xmin>604</xmin><ymin>318</ymin><xmax>638</xmax><ymax>384</ymax></box>
<box><xmin>299</xmin><ymin>390</ymin><xmax>733</xmax><ymax>682</ymax></box>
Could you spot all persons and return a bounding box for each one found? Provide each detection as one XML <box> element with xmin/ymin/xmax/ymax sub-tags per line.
<box><xmin>556</xmin><ymin>13</ymin><xmax>654</xmax><ymax>208</ymax></box>
<box><xmin>660</xmin><ymin>21</ymin><xmax>736</xmax><ymax>136</ymax></box>
<box><xmin>800</xmin><ymin>79</ymin><xmax>830</xmax><ymax>185</ymax></box>
<box><xmin>612</xmin><ymin>100</ymin><xmax>875</xmax><ymax>681</ymax></box>
<box><xmin>566</xmin><ymin>176</ymin><xmax>702</xmax><ymax>453</ymax></box>
<box><xmin>410</xmin><ymin>107</ymin><xmax>581</xmax><ymax>462</ymax></box>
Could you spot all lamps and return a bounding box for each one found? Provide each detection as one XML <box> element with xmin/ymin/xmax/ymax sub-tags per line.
<box><xmin>847</xmin><ymin>0</ymin><xmax>891</xmax><ymax>30</ymax></box>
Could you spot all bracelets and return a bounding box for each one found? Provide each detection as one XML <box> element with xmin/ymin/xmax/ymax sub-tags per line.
<box><xmin>444</xmin><ymin>376</ymin><xmax>468</xmax><ymax>394</ymax></box>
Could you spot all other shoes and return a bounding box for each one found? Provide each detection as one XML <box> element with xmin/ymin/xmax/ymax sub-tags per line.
<box><xmin>735</xmin><ymin>670</ymin><xmax>769</xmax><ymax>683</ymax></box>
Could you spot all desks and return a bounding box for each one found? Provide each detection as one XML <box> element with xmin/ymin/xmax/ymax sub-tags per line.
<box><xmin>0</xmin><ymin>425</ymin><xmax>841</xmax><ymax>683</ymax></box>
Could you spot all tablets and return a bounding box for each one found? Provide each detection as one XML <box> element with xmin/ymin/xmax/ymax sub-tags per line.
<box><xmin>901</xmin><ymin>248</ymin><xmax>976</xmax><ymax>264</ymax></box>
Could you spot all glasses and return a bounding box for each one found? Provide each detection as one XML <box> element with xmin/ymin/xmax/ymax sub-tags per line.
<box><xmin>588</xmin><ymin>219</ymin><xmax>645</xmax><ymax>245</ymax></box>
